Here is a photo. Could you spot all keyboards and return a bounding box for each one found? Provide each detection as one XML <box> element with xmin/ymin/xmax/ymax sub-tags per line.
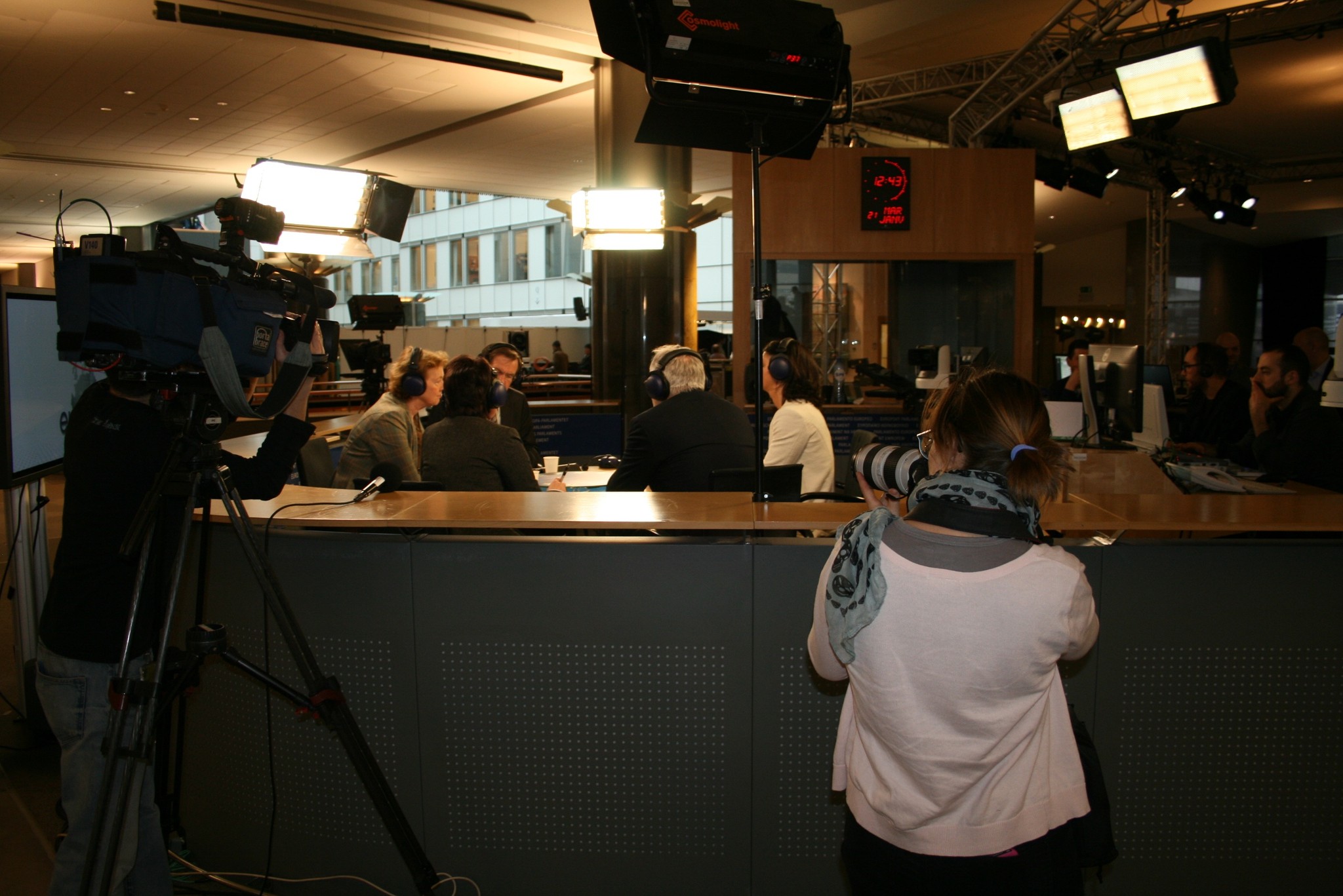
<box><xmin>1164</xmin><ymin>446</ymin><xmax>1267</xmax><ymax>478</ymax></box>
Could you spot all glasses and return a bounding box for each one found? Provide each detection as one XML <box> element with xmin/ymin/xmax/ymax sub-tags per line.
<box><xmin>1183</xmin><ymin>361</ymin><xmax>1200</xmax><ymax>372</ymax></box>
<box><xmin>493</xmin><ymin>368</ymin><xmax>517</xmax><ymax>381</ymax></box>
<box><xmin>917</xmin><ymin>426</ymin><xmax>962</xmax><ymax>460</ymax></box>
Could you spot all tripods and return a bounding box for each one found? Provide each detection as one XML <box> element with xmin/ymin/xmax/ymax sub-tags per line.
<box><xmin>84</xmin><ymin>378</ymin><xmax>446</xmax><ymax>896</ymax></box>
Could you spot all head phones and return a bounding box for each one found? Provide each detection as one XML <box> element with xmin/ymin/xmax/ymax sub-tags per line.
<box><xmin>399</xmin><ymin>347</ymin><xmax>427</xmax><ymax>396</ymax></box>
<box><xmin>489</xmin><ymin>366</ymin><xmax>507</xmax><ymax>408</ymax></box>
<box><xmin>476</xmin><ymin>342</ymin><xmax>526</xmax><ymax>390</ymax></box>
<box><xmin>769</xmin><ymin>338</ymin><xmax>802</xmax><ymax>380</ymax></box>
<box><xmin>643</xmin><ymin>350</ymin><xmax>714</xmax><ymax>399</ymax></box>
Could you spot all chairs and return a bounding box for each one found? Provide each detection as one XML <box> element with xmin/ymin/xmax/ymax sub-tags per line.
<box><xmin>296</xmin><ymin>437</ymin><xmax>335</xmax><ymax>488</ymax></box>
<box><xmin>798</xmin><ymin>428</ymin><xmax>879</xmax><ymax>537</ymax></box>
<box><xmin>647</xmin><ymin>463</ymin><xmax>803</xmax><ymax>537</ymax></box>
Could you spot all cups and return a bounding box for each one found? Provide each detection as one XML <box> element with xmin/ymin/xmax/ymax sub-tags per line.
<box><xmin>543</xmin><ymin>456</ymin><xmax>560</xmax><ymax>473</ymax></box>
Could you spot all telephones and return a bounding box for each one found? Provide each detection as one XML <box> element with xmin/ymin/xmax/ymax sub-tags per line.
<box><xmin>1165</xmin><ymin>462</ymin><xmax>1248</xmax><ymax>493</ymax></box>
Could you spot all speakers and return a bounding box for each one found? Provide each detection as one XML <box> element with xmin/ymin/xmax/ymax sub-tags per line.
<box><xmin>509</xmin><ymin>330</ymin><xmax>529</xmax><ymax>356</ymax></box>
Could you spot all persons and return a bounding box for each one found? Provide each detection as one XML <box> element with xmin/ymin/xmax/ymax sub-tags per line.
<box><xmin>34</xmin><ymin>311</ymin><xmax>325</xmax><ymax>896</ymax></box>
<box><xmin>333</xmin><ymin>340</ymin><xmax>838</xmax><ymax>538</ymax></box>
<box><xmin>807</xmin><ymin>370</ymin><xmax>1100</xmax><ymax>896</ymax></box>
<box><xmin>1058</xmin><ymin>326</ymin><xmax>1343</xmax><ymax>493</ymax></box>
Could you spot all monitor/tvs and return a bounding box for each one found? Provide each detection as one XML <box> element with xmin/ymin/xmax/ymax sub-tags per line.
<box><xmin>0</xmin><ymin>283</ymin><xmax>107</xmax><ymax>490</ymax></box>
<box><xmin>960</xmin><ymin>347</ymin><xmax>988</xmax><ymax>366</ymax></box>
<box><xmin>1077</xmin><ymin>344</ymin><xmax>1139</xmax><ymax>448</ymax></box>
<box><xmin>1135</xmin><ymin>365</ymin><xmax>1175</xmax><ymax>406</ymax></box>
<box><xmin>913</xmin><ymin>345</ymin><xmax>939</xmax><ymax>378</ymax></box>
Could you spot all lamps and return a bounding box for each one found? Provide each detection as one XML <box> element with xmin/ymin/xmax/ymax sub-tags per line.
<box><xmin>1055</xmin><ymin>73</ymin><xmax>1137</xmax><ymax>151</ymax></box>
<box><xmin>571</xmin><ymin>188</ymin><xmax>669</xmax><ymax>251</ymax></box>
<box><xmin>1090</xmin><ymin>148</ymin><xmax>1121</xmax><ymax>179</ymax></box>
<box><xmin>1115</xmin><ymin>15</ymin><xmax>1237</xmax><ymax>127</ymax></box>
<box><xmin>240</xmin><ymin>156</ymin><xmax>416</xmax><ymax>256</ymax></box>
<box><xmin>1158</xmin><ymin>166</ymin><xmax>1258</xmax><ymax>229</ymax></box>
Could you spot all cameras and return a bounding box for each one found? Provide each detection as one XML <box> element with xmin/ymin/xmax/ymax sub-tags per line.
<box><xmin>848</xmin><ymin>444</ymin><xmax>928</xmax><ymax>497</ymax></box>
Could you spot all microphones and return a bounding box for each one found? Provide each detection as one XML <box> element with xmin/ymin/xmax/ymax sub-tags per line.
<box><xmin>273</xmin><ymin>278</ymin><xmax>336</xmax><ymax>308</ymax></box>
<box><xmin>354</xmin><ymin>461</ymin><xmax>403</xmax><ymax>502</ymax></box>
